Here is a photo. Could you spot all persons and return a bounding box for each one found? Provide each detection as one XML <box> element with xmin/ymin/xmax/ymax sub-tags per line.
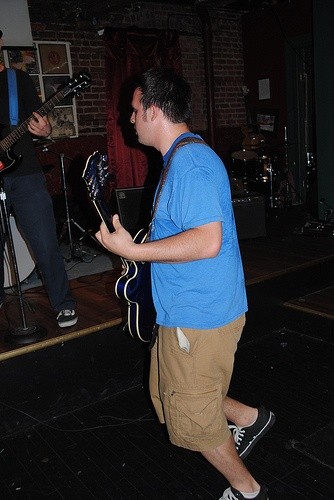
<box><xmin>94</xmin><ymin>68</ymin><xmax>275</xmax><ymax>500</ymax></box>
<box><xmin>0</xmin><ymin>31</ymin><xmax>78</xmax><ymax>328</ymax></box>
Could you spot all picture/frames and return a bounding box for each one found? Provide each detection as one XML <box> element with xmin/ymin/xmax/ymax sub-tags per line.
<box><xmin>0</xmin><ymin>41</ymin><xmax>80</xmax><ymax>140</ymax></box>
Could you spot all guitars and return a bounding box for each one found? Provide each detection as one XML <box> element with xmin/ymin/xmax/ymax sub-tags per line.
<box><xmin>0</xmin><ymin>71</ymin><xmax>93</xmax><ymax>174</ymax></box>
<box><xmin>82</xmin><ymin>150</ymin><xmax>157</xmax><ymax>344</ymax></box>
<box><xmin>239</xmin><ymin>84</ymin><xmax>268</xmax><ymax>151</ymax></box>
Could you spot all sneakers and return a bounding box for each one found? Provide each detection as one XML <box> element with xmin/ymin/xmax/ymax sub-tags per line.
<box><xmin>229</xmin><ymin>406</ymin><xmax>278</xmax><ymax>461</ymax></box>
<box><xmin>216</xmin><ymin>484</ymin><xmax>271</xmax><ymax>500</ymax></box>
<box><xmin>56</xmin><ymin>309</ymin><xmax>79</xmax><ymax>327</ymax></box>
